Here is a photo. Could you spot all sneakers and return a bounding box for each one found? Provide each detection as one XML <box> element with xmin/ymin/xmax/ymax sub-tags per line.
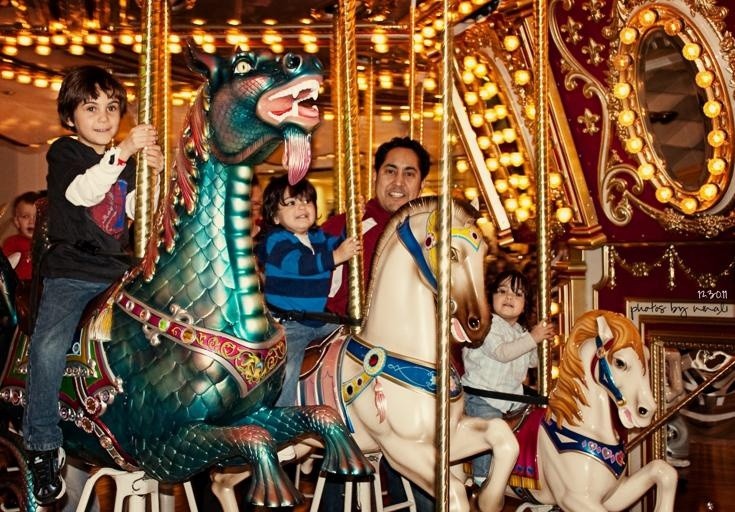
<box><xmin>28</xmin><ymin>446</ymin><xmax>67</xmax><ymax>507</ymax></box>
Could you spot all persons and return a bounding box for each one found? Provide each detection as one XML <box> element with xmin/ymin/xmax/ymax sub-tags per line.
<box><xmin>459</xmin><ymin>270</ymin><xmax>555</xmax><ymax>487</ymax></box>
<box><xmin>252</xmin><ymin>173</ymin><xmax>359</xmax><ymax>461</ymax></box>
<box><xmin>320</xmin><ymin>137</ymin><xmax>430</xmax><ymax>320</ymax></box>
<box><xmin>2</xmin><ymin>191</ymin><xmax>40</xmax><ymax>282</ymax></box>
<box><xmin>23</xmin><ymin>66</ymin><xmax>164</xmax><ymax>507</ymax></box>
